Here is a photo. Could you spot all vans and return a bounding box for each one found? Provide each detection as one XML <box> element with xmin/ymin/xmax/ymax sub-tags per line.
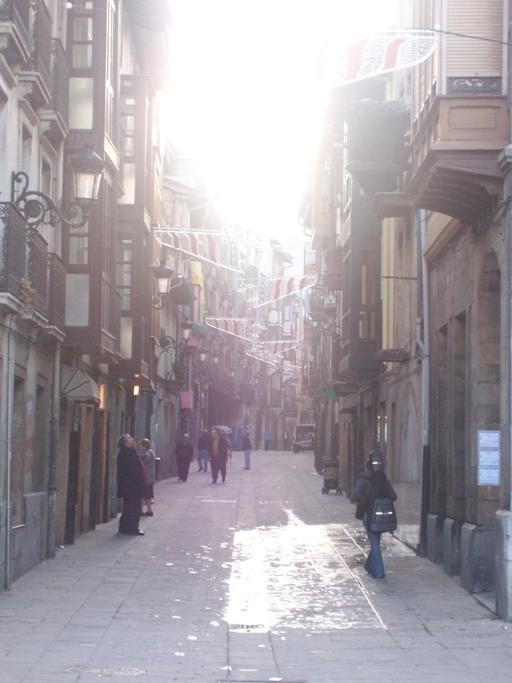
<box><xmin>291</xmin><ymin>422</ymin><xmax>316</xmax><ymax>453</ymax></box>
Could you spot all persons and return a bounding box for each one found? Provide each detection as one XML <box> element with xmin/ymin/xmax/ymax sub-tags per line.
<box><xmin>136</xmin><ymin>438</ymin><xmax>156</xmax><ymax>517</ymax></box>
<box><xmin>350</xmin><ymin>451</ymin><xmax>397</xmax><ymax>579</ymax></box>
<box><xmin>116</xmin><ymin>433</ymin><xmax>150</xmax><ymax>536</ymax></box>
<box><xmin>175</xmin><ymin>433</ymin><xmax>194</xmax><ymax>482</ymax></box>
<box><xmin>242</xmin><ymin>432</ymin><xmax>254</xmax><ymax>470</ymax></box>
<box><xmin>197</xmin><ymin>429</ymin><xmax>232</xmax><ymax>484</ymax></box>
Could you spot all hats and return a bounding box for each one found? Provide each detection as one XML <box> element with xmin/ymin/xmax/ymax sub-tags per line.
<box><xmin>366</xmin><ymin>450</ymin><xmax>385</xmax><ymax>463</ymax></box>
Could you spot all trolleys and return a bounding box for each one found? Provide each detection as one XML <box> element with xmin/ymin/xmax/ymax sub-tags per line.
<box><xmin>320</xmin><ymin>459</ymin><xmax>342</xmax><ymax>495</ymax></box>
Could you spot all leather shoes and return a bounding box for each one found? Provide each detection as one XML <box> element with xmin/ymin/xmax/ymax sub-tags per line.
<box><xmin>142</xmin><ymin>511</ymin><xmax>152</xmax><ymax>516</ymax></box>
<box><xmin>136</xmin><ymin>529</ymin><xmax>145</xmax><ymax>536</ymax></box>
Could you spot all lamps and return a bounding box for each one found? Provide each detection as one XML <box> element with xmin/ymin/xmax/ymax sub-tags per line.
<box><xmin>11</xmin><ymin>144</ymin><xmax>104</xmax><ymax>227</ymax></box>
<box><xmin>151</xmin><ymin>256</ymin><xmax>174</xmax><ymax>309</ymax></box>
<box><xmin>150</xmin><ymin>318</ymin><xmax>191</xmax><ymax>352</ymax></box>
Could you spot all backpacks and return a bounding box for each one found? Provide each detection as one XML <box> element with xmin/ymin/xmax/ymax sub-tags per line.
<box><xmin>359</xmin><ymin>476</ymin><xmax>398</xmax><ymax>533</ymax></box>
